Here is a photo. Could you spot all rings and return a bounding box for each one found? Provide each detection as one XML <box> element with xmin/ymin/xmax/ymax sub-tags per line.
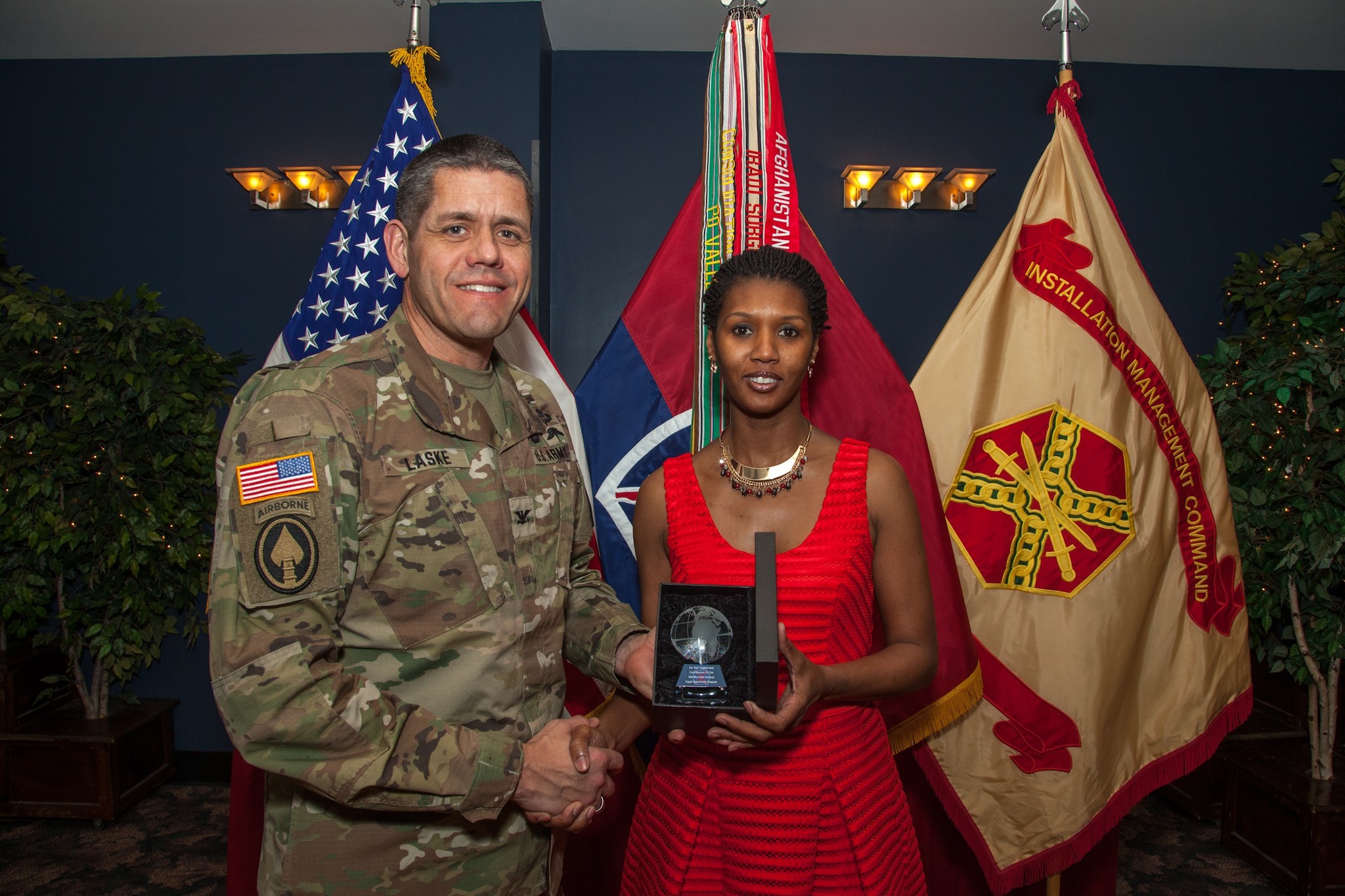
<box><xmin>596</xmin><ymin>796</ymin><xmax>606</xmax><ymax>814</ymax></box>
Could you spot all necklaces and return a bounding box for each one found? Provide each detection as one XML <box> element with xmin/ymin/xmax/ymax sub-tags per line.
<box><xmin>717</xmin><ymin>418</ymin><xmax>813</xmax><ymax>499</ymax></box>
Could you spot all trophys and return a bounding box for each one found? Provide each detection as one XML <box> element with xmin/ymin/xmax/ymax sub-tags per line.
<box><xmin>670</xmin><ymin>605</ymin><xmax>733</xmax><ymax>699</ymax></box>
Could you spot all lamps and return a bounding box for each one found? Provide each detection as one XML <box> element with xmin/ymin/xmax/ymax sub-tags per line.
<box><xmin>332</xmin><ymin>166</ymin><xmax>362</xmax><ymax>186</ymax></box>
<box><xmin>278</xmin><ymin>166</ymin><xmax>333</xmax><ymax>208</ymax></box>
<box><xmin>224</xmin><ymin>166</ymin><xmax>282</xmax><ymax>209</ymax></box>
<box><xmin>840</xmin><ymin>166</ymin><xmax>889</xmax><ymax>207</ymax></box>
<box><xmin>943</xmin><ymin>168</ymin><xmax>997</xmax><ymax>209</ymax></box>
<box><xmin>893</xmin><ymin>166</ymin><xmax>943</xmax><ymax>209</ymax></box>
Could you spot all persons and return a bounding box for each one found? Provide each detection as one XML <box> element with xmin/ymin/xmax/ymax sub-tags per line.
<box><xmin>532</xmin><ymin>242</ymin><xmax>939</xmax><ymax>896</ymax></box>
<box><xmin>209</xmin><ymin>132</ymin><xmax>703</xmax><ymax>896</ymax></box>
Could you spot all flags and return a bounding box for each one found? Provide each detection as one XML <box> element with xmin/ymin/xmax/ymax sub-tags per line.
<box><xmin>576</xmin><ymin>17</ymin><xmax>981</xmax><ymax>776</ymax></box>
<box><xmin>906</xmin><ymin>84</ymin><xmax>1254</xmax><ymax>896</ymax></box>
<box><xmin>217</xmin><ymin>50</ymin><xmax>604</xmax><ymax>896</ymax></box>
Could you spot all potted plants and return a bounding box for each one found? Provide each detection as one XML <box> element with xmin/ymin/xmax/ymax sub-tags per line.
<box><xmin>0</xmin><ymin>263</ymin><xmax>251</xmax><ymax>827</ymax></box>
<box><xmin>1191</xmin><ymin>159</ymin><xmax>1345</xmax><ymax>896</ymax></box>
